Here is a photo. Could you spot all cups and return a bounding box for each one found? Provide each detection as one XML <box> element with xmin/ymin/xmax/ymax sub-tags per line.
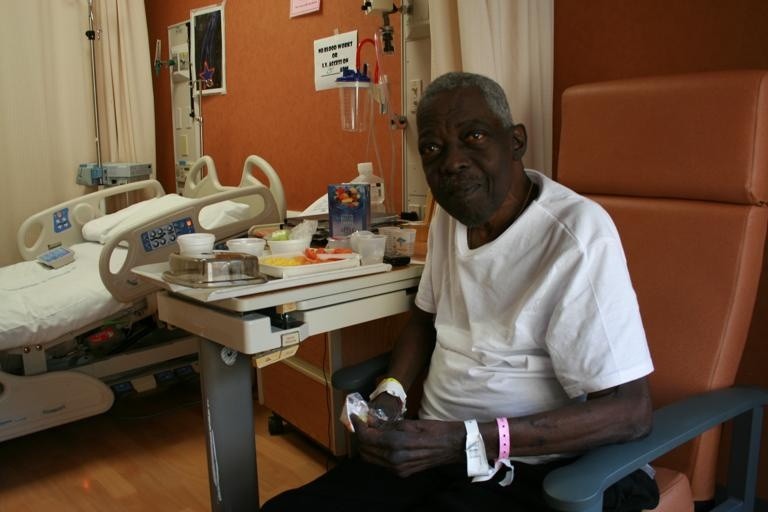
<box><xmin>380</xmin><ymin>223</ymin><xmax>417</xmax><ymax>258</ymax></box>
<box><xmin>226</xmin><ymin>237</ymin><xmax>266</xmax><ymax>259</ymax></box>
<box><xmin>356</xmin><ymin>235</ymin><xmax>387</xmax><ymax>266</ymax></box>
<box><xmin>267</xmin><ymin>238</ymin><xmax>306</xmax><ymax>255</ymax></box>
<box><xmin>176</xmin><ymin>233</ymin><xmax>215</xmax><ymax>257</ymax></box>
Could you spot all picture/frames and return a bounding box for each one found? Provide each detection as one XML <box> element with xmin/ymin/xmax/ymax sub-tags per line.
<box><xmin>188</xmin><ymin>6</ymin><xmax>226</xmax><ymax>95</ymax></box>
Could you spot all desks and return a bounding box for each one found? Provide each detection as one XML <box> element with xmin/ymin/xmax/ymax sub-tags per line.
<box><xmin>133</xmin><ymin>260</ymin><xmax>428</xmax><ymax>512</ymax></box>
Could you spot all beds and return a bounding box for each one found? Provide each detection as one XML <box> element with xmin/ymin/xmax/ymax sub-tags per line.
<box><xmin>0</xmin><ymin>154</ymin><xmax>287</xmax><ymax>443</ymax></box>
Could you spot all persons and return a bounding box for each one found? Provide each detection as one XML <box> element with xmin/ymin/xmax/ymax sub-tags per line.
<box><xmin>269</xmin><ymin>72</ymin><xmax>661</xmax><ymax>511</ymax></box>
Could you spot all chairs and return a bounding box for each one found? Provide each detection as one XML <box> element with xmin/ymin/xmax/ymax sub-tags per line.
<box><xmin>329</xmin><ymin>68</ymin><xmax>768</xmax><ymax>512</ymax></box>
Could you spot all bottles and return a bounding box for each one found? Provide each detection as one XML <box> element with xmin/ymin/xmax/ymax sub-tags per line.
<box><xmin>335</xmin><ymin>63</ymin><xmax>373</xmax><ymax>131</ymax></box>
<box><xmin>352</xmin><ymin>163</ymin><xmax>385</xmax><ymax>221</ymax></box>
